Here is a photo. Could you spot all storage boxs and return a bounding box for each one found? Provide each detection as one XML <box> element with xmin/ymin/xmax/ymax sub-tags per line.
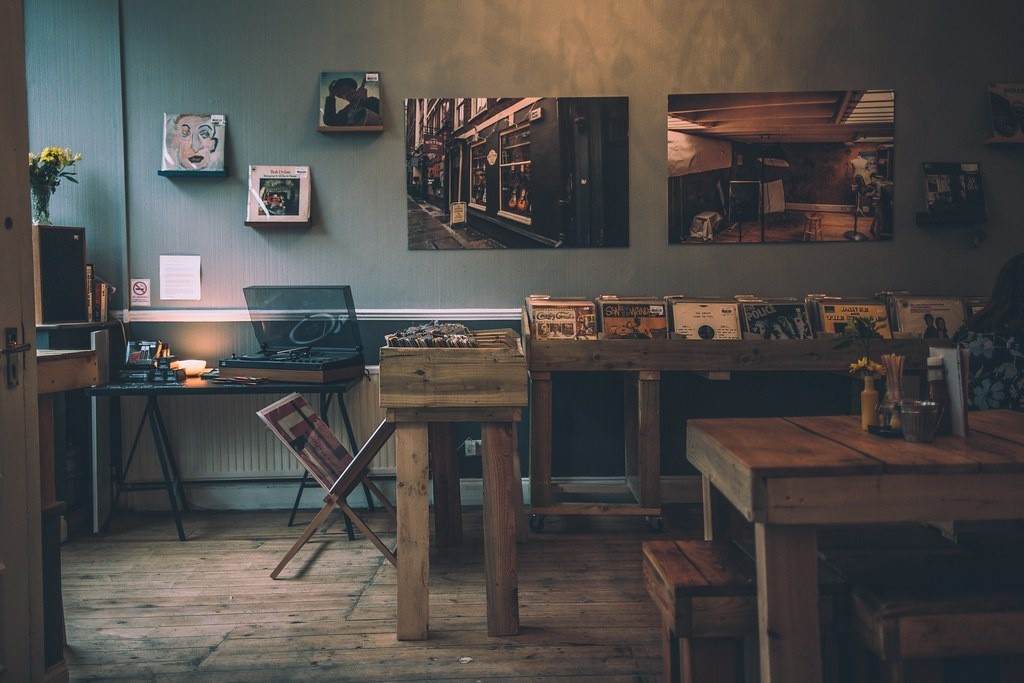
<box><xmin>379</xmin><ymin>328</ymin><xmax>528</xmax><ymax>407</ymax></box>
<box><xmin>165</xmin><ymin>368</ymin><xmax>187</xmax><ymax>382</ymax></box>
<box><xmin>157</xmin><ymin>356</ymin><xmax>176</xmax><ymax>369</ymax></box>
<box><xmin>117</xmin><ymin>370</ymin><xmax>155</xmax><ymax>382</ymax></box>
<box><xmin>219</xmin><ymin>285</ymin><xmax>365</xmax><ymax>383</ymax></box>
<box><xmin>153</xmin><ymin>369</ymin><xmax>165</xmax><ymax>381</ymax></box>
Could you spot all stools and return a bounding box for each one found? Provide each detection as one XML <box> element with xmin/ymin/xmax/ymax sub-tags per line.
<box><xmin>641</xmin><ymin>539</ymin><xmax>1024</xmax><ymax>683</ymax></box>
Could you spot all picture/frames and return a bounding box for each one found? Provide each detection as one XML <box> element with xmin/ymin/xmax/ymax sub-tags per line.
<box><xmin>247</xmin><ymin>164</ymin><xmax>312</xmax><ymax>222</ymax></box>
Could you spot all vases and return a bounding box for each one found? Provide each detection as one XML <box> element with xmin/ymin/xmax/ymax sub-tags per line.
<box><xmin>30</xmin><ymin>186</ymin><xmax>55</xmax><ymax>226</ymax></box>
<box><xmin>861</xmin><ymin>376</ymin><xmax>880</xmax><ymax>431</ymax></box>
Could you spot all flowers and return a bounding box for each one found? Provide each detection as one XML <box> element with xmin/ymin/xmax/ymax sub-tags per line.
<box><xmin>29</xmin><ymin>146</ymin><xmax>83</xmax><ymax>219</ymax></box>
<box><xmin>832</xmin><ymin>316</ymin><xmax>888</xmax><ymax>377</ymax></box>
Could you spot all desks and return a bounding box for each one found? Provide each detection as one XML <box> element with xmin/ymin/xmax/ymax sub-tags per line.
<box><xmin>35</xmin><ymin>329</ymin><xmax>112</xmax><ymax>535</ymax></box>
<box><xmin>84</xmin><ymin>376</ymin><xmax>375</xmax><ymax>542</ymax></box>
<box><xmin>386</xmin><ymin>407</ymin><xmax>529</xmax><ymax>643</ymax></box>
<box><xmin>522</xmin><ymin>331</ymin><xmax>952</xmax><ymax>533</ymax></box>
<box><xmin>36</xmin><ymin>319</ymin><xmax>121</xmax><ymax>538</ymax></box>
<box><xmin>684</xmin><ymin>410</ymin><xmax>1024</xmax><ymax>683</ymax></box>
